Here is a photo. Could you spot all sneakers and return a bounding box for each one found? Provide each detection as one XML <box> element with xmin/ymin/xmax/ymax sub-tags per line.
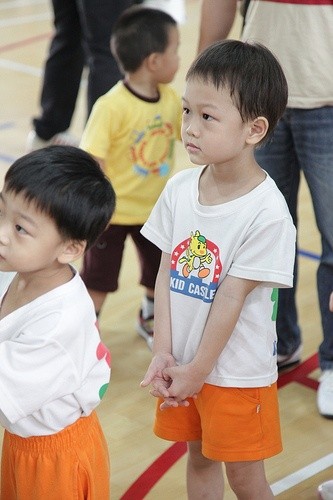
<box><xmin>276</xmin><ymin>344</ymin><xmax>301</xmax><ymax>369</ymax></box>
<box><xmin>135</xmin><ymin>309</ymin><xmax>154</xmax><ymax>349</ymax></box>
<box><xmin>28</xmin><ymin>132</ymin><xmax>78</xmax><ymax>153</ymax></box>
<box><xmin>316</xmin><ymin>368</ymin><xmax>333</xmax><ymax>417</ymax></box>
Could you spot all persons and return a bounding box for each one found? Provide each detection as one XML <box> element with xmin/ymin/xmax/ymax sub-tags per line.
<box><xmin>26</xmin><ymin>0</ymin><xmax>143</xmax><ymax>153</ymax></box>
<box><xmin>78</xmin><ymin>7</ymin><xmax>183</xmax><ymax>352</ymax></box>
<box><xmin>196</xmin><ymin>0</ymin><xmax>333</xmax><ymax>420</ymax></box>
<box><xmin>0</xmin><ymin>145</ymin><xmax>116</xmax><ymax>500</ymax></box>
<box><xmin>140</xmin><ymin>39</ymin><xmax>296</xmax><ymax>500</ymax></box>
